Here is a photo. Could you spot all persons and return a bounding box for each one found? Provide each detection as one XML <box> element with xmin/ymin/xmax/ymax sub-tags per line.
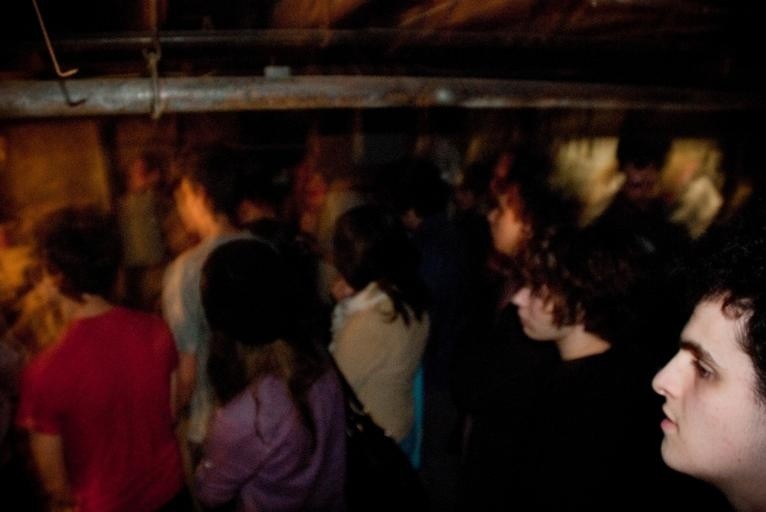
<box><xmin>0</xmin><ymin>117</ymin><xmax>766</xmax><ymax>512</ymax></box>
